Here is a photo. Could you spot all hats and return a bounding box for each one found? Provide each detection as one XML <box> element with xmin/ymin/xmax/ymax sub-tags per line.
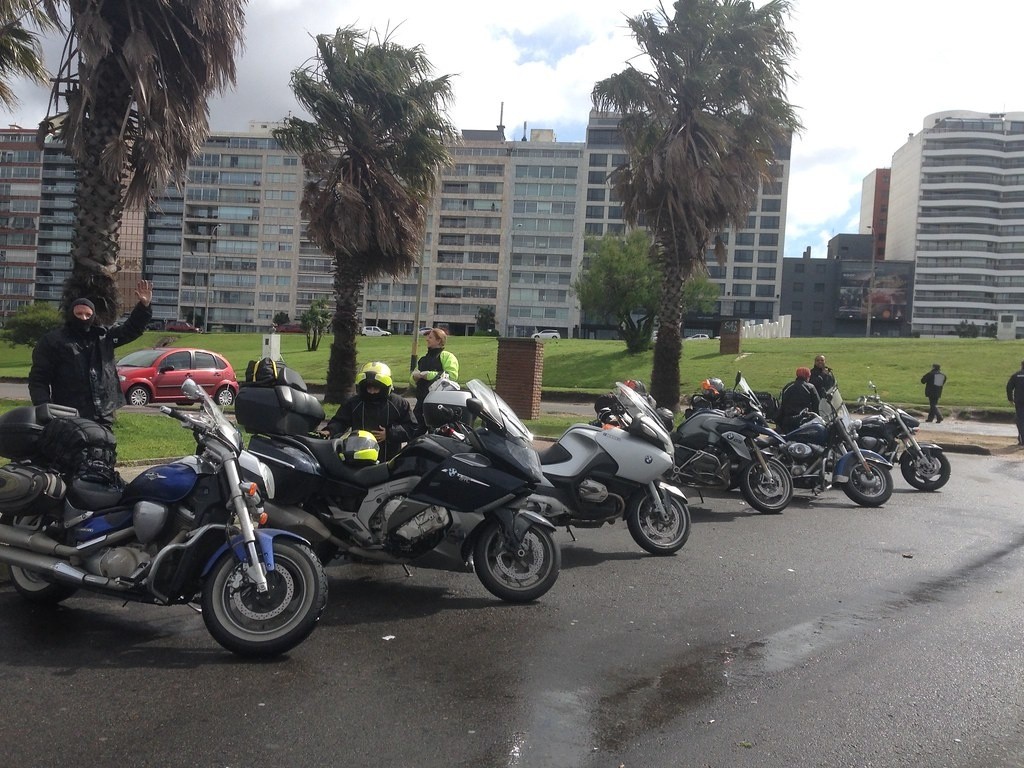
<box><xmin>68</xmin><ymin>297</ymin><xmax>96</xmax><ymax>333</ymax></box>
<box><xmin>796</xmin><ymin>367</ymin><xmax>812</xmax><ymax>378</ymax></box>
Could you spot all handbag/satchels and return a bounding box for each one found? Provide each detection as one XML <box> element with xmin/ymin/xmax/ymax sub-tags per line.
<box><xmin>245</xmin><ymin>356</ymin><xmax>288</xmax><ymax>385</ymax></box>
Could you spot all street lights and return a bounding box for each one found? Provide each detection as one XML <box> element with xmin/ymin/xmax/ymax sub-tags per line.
<box><xmin>505</xmin><ymin>223</ymin><xmax>522</xmax><ymax>337</ymax></box>
<box><xmin>866</xmin><ymin>225</ymin><xmax>876</xmax><ymax>338</ymax></box>
<box><xmin>202</xmin><ymin>224</ymin><xmax>223</xmax><ymax>334</ymax></box>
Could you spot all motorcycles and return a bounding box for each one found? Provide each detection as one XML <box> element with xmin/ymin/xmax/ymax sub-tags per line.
<box><xmin>754</xmin><ymin>380</ymin><xmax>951</xmax><ymax>492</ymax></box>
<box><xmin>235</xmin><ymin>379</ymin><xmax>566</xmax><ymax>606</ymax></box>
<box><xmin>0</xmin><ymin>377</ymin><xmax>329</xmax><ymax>659</ymax></box>
<box><xmin>752</xmin><ymin>376</ymin><xmax>894</xmax><ymax>508</ymax></box>
<box><xmin>616</xmin><ymin>371</ymin><xmax>795</xmax><ymax>513</ymax></box>
<box><xmin>422</xmin><ymin>382</ymin><xmax>694</xmax><ymax>557</ymax></box>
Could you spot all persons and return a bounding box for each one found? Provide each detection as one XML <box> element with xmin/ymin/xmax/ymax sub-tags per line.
<box><xmin>314</xmin><ymin>362</ymin><xmax>417</xmax><ymax>464</ymax></box>
<box><xmin>411</xmin><ymin>328</ymin><xmax>459</xmax><ymax>435</ymax></box>
<box><xmin>809</xmin><ymin>354</ymin><xmax>834</xmax><ymax>399</ymax></box>
<box><xmin>779</xmin><ymin>366</ymin><xmax>821</xmax><ymax>432</ymax></box>
<box><xmin>921</xmin><ymin>364</ymin><xmax>946</xmax><ymax>423</ymax></box>
<box><xmin>29</xmin><ymin>279</ymin><xmax>154</xmax><ymax>430</ymax></box>
<box><xmin>1007</xmin><ymin>359</ymin><xmax>1024</xmax><ymax>445</ymax></box>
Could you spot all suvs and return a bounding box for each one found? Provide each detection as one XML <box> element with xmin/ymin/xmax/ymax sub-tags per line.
<box><xmin>361</xmin><ymin>326</ymin><xmax>391</xmax><ymax>337</ymax></box>
<box><xmin>166</xmin><ymin>320</ymin><xmax>201</xmax><ymax>333</ymax></box>
<box><xmin>531</xmin><ymin>330</ymin><xmax>561</xmax><ymax>340</ymax></box>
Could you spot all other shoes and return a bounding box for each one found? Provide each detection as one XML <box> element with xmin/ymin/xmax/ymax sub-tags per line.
<box><xmin>936</xmin><ymin>417</ymin><xmax>943</xmax><ymax>423</ymax></box>
<box><xmin>925</xmin><ymin>419</ymin><xmax>932</xmax><ymax>422</ymax></box>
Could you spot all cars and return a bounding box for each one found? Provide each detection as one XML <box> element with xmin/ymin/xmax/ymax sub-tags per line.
<box><xmin>687</xmin><ymin>333</ymin><xmax>709</xmax><ymax>340</ymax></box>
<box><xmin>145</xmin><ymin>321</ymin><xmax>164</xmax><ymax>331</ymax></box>
<box><xmin>277</xmin><ymin>323</ymin><xmax>303</xmax><ymax>333</ymax></box>
<box><xmin>418</xmin><ymin>327</ymin><xmax>449</xmax><ymax>336</ymax></box>
<box><xmin>116</xmin><ymin>347</ymin><xmax>239</xmax><ymax>408</ymax></box>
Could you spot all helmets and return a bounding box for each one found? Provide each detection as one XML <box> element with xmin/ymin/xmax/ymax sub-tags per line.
<box><xmin>335</xmin><ymin>429</ymin><xmax>380</xmax><ymax>466</ymax></box>
<box><xmin>710</xmin><ymin>378</ymin><xmax>724</xmax><ymax>392</ymax></box>
<box><xmin>355</xmin><ymin>361</ymin><xmax>394</xmax><ymax>404</ymax></box>
